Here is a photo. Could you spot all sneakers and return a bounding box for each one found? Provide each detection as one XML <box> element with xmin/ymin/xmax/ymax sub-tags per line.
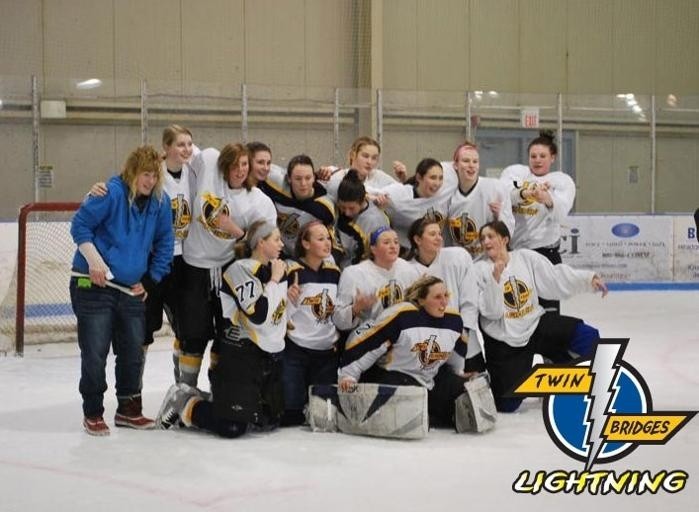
<box><xmin>81</xmin><ymin>415</ymin><xmax>113</xmax><ymax>436</ymax></box>
<box><xmin>114</xmin><ymin>410</ymin><xmax>157</xmax><ymax>430</ymax></box>
<box><xmin>159</xmin><ymin>387</ymin><xmax>190</xmax><ymax>430</ymax></box>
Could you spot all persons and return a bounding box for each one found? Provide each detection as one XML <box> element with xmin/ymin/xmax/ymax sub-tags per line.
<box><xmin>70</xmin><ymin>124</ymin><xmax>609</xmax><ymax>438</ymax></box>
